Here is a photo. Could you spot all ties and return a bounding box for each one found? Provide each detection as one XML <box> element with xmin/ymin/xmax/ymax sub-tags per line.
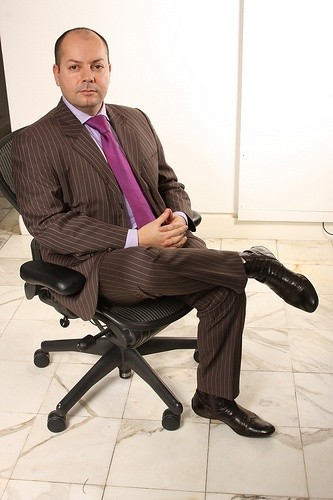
<box><xmin>85</xmin><ymin>115</ymin><xmax>156</xmax><ymax>230</ymax></box>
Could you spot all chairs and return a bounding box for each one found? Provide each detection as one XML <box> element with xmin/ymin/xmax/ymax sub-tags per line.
<box><xmin>0</xmin><ymin>125</ymin><xmax>201</xmax><ymax>433</ymax></box>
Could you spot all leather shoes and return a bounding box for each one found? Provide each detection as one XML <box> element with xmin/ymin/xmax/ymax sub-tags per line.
<box><xmin>192</xmin><ymin>390</ymin><xmax>275</xmax><ymax>437</ymax></box>
<box><xmin>244</xmin><ymin>246</ymin><xmax>319</xmax><ymax>313</ymax></box>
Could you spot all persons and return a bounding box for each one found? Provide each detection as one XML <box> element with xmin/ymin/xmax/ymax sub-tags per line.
<box><xmin>11</xmin><ymin>27</ymin><xmax>318</xmax><ymax>439</ymax></box>
